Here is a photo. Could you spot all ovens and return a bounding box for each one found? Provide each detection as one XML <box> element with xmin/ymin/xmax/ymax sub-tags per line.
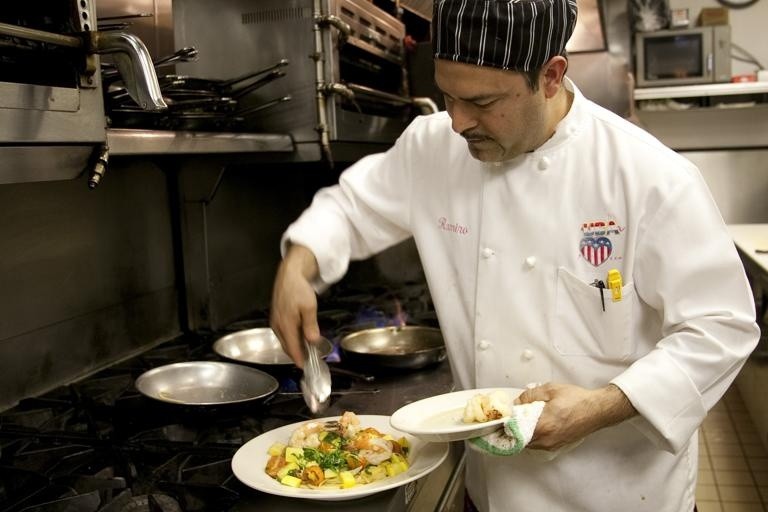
<box><xmin>2</xmin><ymin>0</ymin><xmax>168</xmax><ymax>147</ymax></box>
<box><xmin>170</xmin><ymin>0</ymin><xmax>437</xmax><ymax>144</ymax></box>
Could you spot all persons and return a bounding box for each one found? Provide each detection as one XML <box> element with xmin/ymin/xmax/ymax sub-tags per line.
<box><xmin>269</xmin><ymin>1</ymin><xmax>761</xmax><ymax>512</ymax></box>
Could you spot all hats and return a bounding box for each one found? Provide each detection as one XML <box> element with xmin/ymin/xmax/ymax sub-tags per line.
<box><xmin>431</xmin><ymin>1</ymin><xmax>578</xmax><ymax>73</ymax></box>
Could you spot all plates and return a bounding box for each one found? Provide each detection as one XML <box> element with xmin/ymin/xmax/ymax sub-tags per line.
<box><xmin>390</xmin><ymin>389</ymin><xmax>546</xmax><ymax>444</ymax></box>
<box><xmin>230</xmin><ymin>413</ymin><xmax>452</xmax><ymax>502</ymax></box>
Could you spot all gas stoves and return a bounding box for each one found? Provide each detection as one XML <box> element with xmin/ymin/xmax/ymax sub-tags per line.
<box><xmin>0</xmin><ymin>291</ymin><xmax>469</xmax><ymax>512</ymax></box>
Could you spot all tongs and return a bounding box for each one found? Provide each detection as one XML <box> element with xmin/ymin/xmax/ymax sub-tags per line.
<box><xmin>298</xmin><ymin>333</ymin><xmax>333</xmax><ymax>412</ymax></box>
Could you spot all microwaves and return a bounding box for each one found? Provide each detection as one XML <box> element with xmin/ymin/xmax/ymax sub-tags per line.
<box><xmin>634</xmin><ymin>26</ymin><xmax>732</xmax><ymax>88</ymax></box>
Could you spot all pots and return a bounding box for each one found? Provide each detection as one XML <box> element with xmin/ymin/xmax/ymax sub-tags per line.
<box><xmin>339</xmin><ymin>323</ymin><xmax>448</xmax><ymax>375</ymax></box>
<box><xmin>96</xmin><ymin>11</ymin><xmax>295</xmax><ymax>132</ymax></box>
<box><xmin>136</xmin><ymin>358</ymin><xmax>383</xmax><ymax>411</ymax></box>
<box><xmin>210</xmin><ymin>324</ymin><xmax>376</xmax><ymax>384</ymax></box>
